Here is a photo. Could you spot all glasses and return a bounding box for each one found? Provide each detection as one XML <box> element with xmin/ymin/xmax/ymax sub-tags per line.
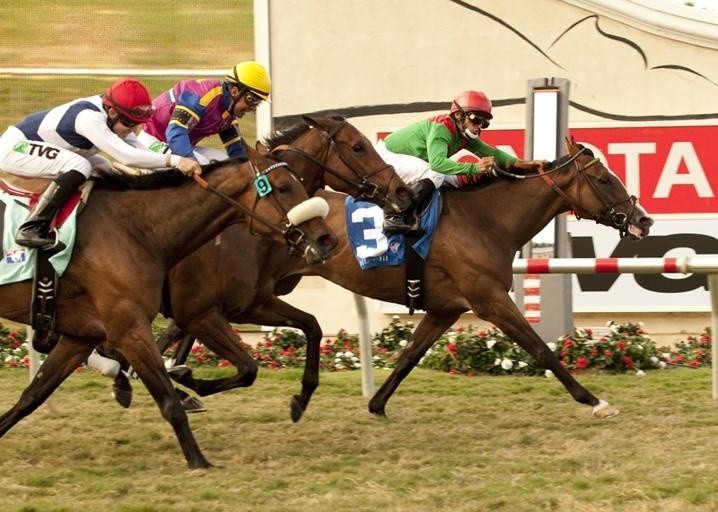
<box><xmin>469</xmin><ymin>114</ymin><xmax>490</xmax><ymax>129</ymax></box>
<box><xmin>245</xmin><ymin>95</ymin><xmax>262</xmax><ymax>107</ymax></box>
<box><xmin>118</xmin><ymin>113</ymin><xmax>146</xmax><ymax>127</ymax></box>
<box><xmin>131</xmin><ymin>105</ymin><xmax>152</xmax><ymax>118</ymax></box>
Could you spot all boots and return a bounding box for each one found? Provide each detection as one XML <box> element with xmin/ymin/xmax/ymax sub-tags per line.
<box><xmin>383</xmin><ymin>178</ymin><xmax>436</xmax><ymax>237</ymax></box>
<box><xmin>15</xmin><ymin>180</ymin><xmax>76</xmax><ymax>250</ymax></box>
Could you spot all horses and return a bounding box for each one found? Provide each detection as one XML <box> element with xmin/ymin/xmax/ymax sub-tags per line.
<box><xmin>0</xmin><ymin>136</ymin><xmax>339</xmax><ymax>470</ymax></box>
<box><xmin>155</xmin><ymin>134</ymin><xmax>655</xmax><ymax>423</ymax></box>
<box><xmin>158</xmin><ymin>111</ymin><xmax>414</xmax><ymax>423</ymax></box>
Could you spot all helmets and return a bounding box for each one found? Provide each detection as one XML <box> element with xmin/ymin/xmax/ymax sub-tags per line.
<box><xmin>225</xmin><ymin>61</ymin><xmax>270</xmax><ymax>103</ymax></box>
<box><xmin>450</xmin><ymin>91</ymin><xmax>493</xmax><ymax>119</ymax></box>
<box><xmin>102</xmin><ymin>78</ymin><xmax>155</xmax><ymax>122</ymax></box>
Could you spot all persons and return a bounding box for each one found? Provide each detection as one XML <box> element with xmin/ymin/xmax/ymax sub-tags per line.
<box><xmin>374</xmin><ymin>90</ymin><xmax>548</xmax><ymax>231</ymax></box>
<box><xmin>143</xmin><ymin>60</ymin><xmax>271</xmax><ymax>171</ymax></box>
<box><xmin>0</xmin><ymin>78</ymin><xmax>202</xmax><ymax>247</ymax></box>
